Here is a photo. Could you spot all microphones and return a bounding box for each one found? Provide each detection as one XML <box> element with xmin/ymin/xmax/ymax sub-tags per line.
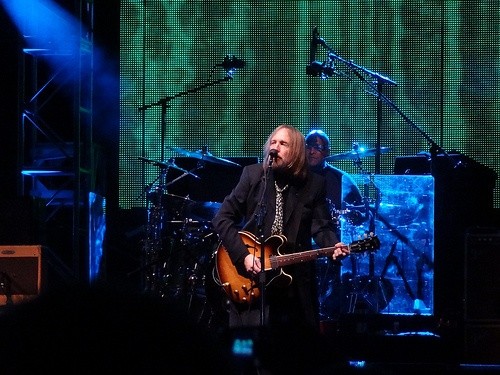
<box><xmin>0</xmin><ymin>272</ymin><xmax>9</xmax><ymax>289</ymax></box>
<box><xmin>381</xmin><ymin>241</ymin><xmax>397</xmax><ymax>275</ymax></box>
<box><xmin>310</xmin><ymin>26</ymin><xmax>318</xmax><ymax>63</ymax></box>
<box><xmin>268</xmin><ymin>149</ymin><xmax>278</xmax><ymax>157</ymax></box>
<box><xmin>310</xmin><ymin>61</ymin><xmax>341</xmax><ymax>77</ymax></box>
<box><xmin>215</xmin><ymin>60</ymin><xmax>245</xmax><ymax>69</ymax></box>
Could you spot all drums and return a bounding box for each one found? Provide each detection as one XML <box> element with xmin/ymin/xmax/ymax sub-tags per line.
<box><xmin>161</xmin><ymin>220</ymin><xmax>211</xmax><ymax>295</ymax></box>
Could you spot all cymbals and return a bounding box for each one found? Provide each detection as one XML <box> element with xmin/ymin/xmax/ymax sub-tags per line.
<box><xmin>168</xmin><ymin>145</ymin><xmax>244</xmax><ymax>169</ymax></box>
<box><xmin>136</xmin><ymin>156</ymin><xmax>203</xmax><ymax>181</ymax></box>
<box><xmin>323</xmin><ymin>145</ymin><xmax>395</xmax><ymax>162</ymax></box>
<box><xmin>148</xmin><ymin>190</ymin><xmax>208</xmax><ymax>215</ymax></box>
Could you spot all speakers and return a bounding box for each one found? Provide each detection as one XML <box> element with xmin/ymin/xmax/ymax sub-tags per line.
<box><xmin>461</xmin><ymin>233</ymin><xmax>500</xmax><ymax>324</ymax></box>
<box><xmin>0</xmin><ymin>245</ymin><xmax>42</xmax><ymax>306</ymax></box>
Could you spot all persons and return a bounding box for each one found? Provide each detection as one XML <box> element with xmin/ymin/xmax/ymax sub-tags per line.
<box><xmin>252</xmin><ymin>318</ymin><xmax>324</xmax><ymax>375</ymax></box>
<box><xmin>302</xmin><ymin>129</ymin><xmax>346</xmax><ymax>246</ymax></box>
<box><xmin>211</xmin><ymin>123</ymin><xmax>351</xmax><ymax>331</ymax></box>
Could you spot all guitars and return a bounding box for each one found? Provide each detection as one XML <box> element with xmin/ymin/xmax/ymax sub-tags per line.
<box><xmin>216</xmin><ymin>231</ymin><xmax>383</xmax><ymax>306</ymax></box>
